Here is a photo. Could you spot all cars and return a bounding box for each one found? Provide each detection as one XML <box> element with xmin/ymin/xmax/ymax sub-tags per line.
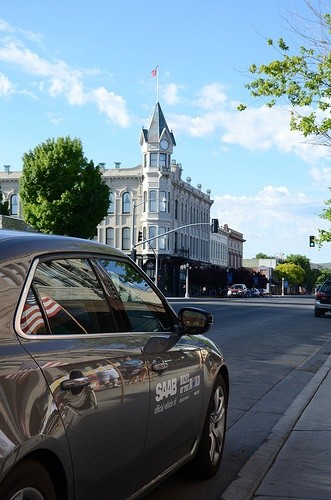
<box><xmin>246</xmin><ymin>288</ymin><xmax>268</xmax><ymax>298</ymax></box>
<box><xmin>0</xmin><ymin>228</ymin><xmax>230</xmax><ymax>500</ymax></box>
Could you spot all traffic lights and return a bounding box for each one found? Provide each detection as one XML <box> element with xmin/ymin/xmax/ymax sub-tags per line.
<box><xmin>309</xmin><ymin>236</ymin><xmax>315</xmax><ymax>247</ymax></box>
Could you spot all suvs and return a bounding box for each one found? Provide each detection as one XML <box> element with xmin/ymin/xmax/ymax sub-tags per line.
<box><xmin>227</xmin><ymin>284</ymin><xmax>248</xmax><ymax>298</ymax></box>
<box><xmin>314</xmin><ymin>278</ymin><xmax>331</xmax><ymax>318</ymax></box>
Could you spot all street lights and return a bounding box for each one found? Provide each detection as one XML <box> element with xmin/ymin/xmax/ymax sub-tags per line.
<box><xmin>185</xmin><ymin>263</ymin><xmax>190</xmax><ymax>299</ymax></box>
